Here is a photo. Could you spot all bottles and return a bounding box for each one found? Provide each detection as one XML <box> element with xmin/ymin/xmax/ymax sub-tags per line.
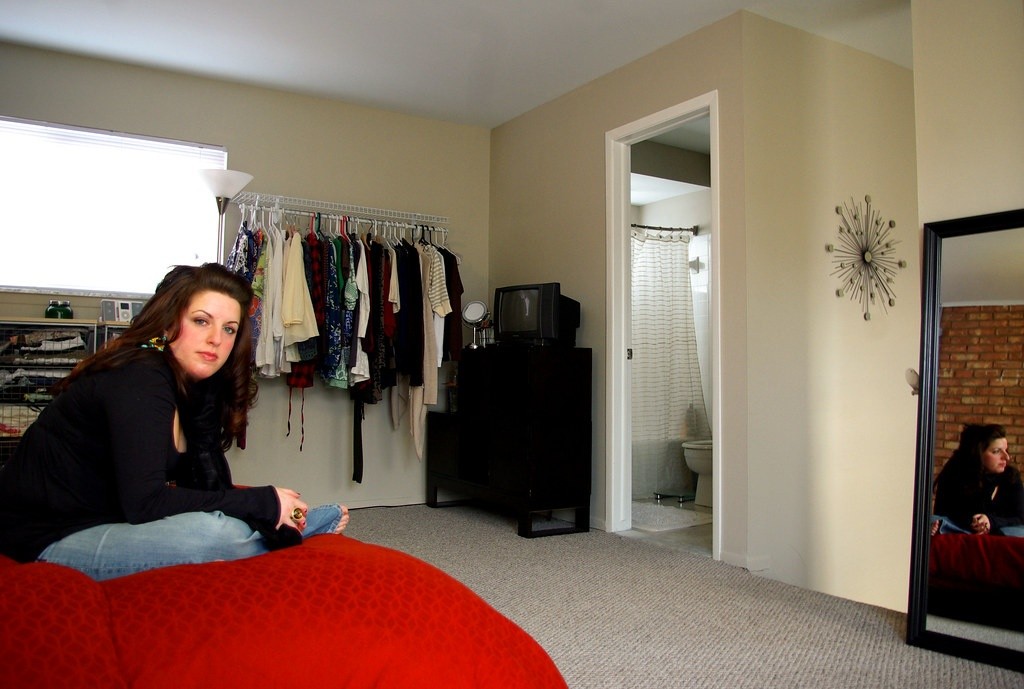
<box><xmin>45</xmin><ymin>300</ymin><xmax>73</xmax><ymax>319</ymax></box>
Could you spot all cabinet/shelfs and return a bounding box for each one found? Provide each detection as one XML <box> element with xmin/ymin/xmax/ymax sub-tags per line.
<box><xmin>0</xmin><ymin>318</ymin><xmax>136</xmax><ymax>448</ymax></box>
<box><xmin>427</xmin><ymin>342</ymin><xmax>594</xmax><ymax>537</ymax></box>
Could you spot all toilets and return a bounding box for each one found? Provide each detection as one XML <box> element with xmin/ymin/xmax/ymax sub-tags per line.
<box><xmin>682</xmin><ymin>439</ymin><xmax>712</xmax><ymax>508</ymax></box>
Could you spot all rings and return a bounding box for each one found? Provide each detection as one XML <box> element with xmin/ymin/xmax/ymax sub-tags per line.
<box><xmin>985</xmin><ymin>524</ymin><xmax>987</xmax><ymax>528</ymax></box>
<box><xmin>291</xmin><ymin>506</ymin><xmax>303</xmax><ymax>522</ymax></box>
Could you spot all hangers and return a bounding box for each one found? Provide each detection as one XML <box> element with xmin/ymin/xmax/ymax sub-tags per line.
<box><xmin>238</xmin><ymin>203</ymin><xmax>462</xmax><ymax>265</ymax></box>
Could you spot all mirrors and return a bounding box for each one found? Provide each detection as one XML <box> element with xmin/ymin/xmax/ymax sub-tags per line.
<box><xmin>460</xmin><ymin>301</ymin><xmax>492</xmax><ymax>348</ymax></box>
<box><xmin>906</xmin><ymin>209</ymin><xmax>1024</xmax><ymax>676</ymax></box>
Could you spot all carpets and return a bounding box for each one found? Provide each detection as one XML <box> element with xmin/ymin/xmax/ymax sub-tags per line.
<box><xmin>631</xmin><ymin>500</ymin><xmax>712</xmax><ymax>533</ymax></box>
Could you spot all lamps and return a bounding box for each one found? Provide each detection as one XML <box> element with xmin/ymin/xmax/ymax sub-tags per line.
<box><xmin>199</xmin><ymin>168</ymin><xmax>253</xmax><ymax>264</ymax></box>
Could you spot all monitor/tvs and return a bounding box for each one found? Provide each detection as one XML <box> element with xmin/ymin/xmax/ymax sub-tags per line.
<box><xmin>494</xmin><ymin>282</ymin><xmax>581</xmax><ymax>347</ymax></box>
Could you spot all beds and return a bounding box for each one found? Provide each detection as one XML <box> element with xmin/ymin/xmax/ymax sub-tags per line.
<box><xmin>0</xmin><ymin>550</ymin><xmax>569</xmax><ymax>689</ymax></box>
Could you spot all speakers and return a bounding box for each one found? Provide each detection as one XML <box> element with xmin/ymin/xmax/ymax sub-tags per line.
<box><xmin>101</xmin><ymin>299</ymin><xmax>117</xmax><ymax>322</ymax></box>
<box><xmin>132</xmin><ymin>302</ymin><xmax>144</xmax><ymax>317</ymax></box>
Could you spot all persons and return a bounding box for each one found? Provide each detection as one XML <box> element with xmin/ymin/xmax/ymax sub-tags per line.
<box><xmin>0</xmin><ymin>263</ymin><xmax>350</xmax><ymax>581</ymax></box>
<box><xmin>931</xmin><ymin>423</ymin><xmax>1024</xmax><ymax>536</ymax></box>
